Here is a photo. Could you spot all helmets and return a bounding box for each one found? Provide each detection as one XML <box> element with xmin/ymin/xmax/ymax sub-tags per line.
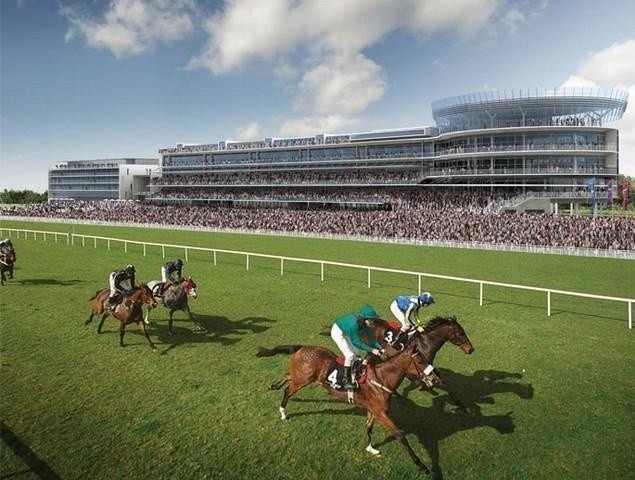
<box><xmin>362</xmin><ymin>308</ymin><xmax>379</xmax><ymax>319</ymax></box>
<box><xmin>126</xmin><ymin>265</ymin><xmax>136</xmax><ymax>273</ymax></box>
<box><xmin>5</xmin><ymin>239</ymin><xmax>11</xmax><ymax>245</ymax></box>
<box><xmin>174</xmin><ymin>259</ymin><xmax>183</xmax><ymax>266</ymax></box>
<box><xmin>420</xmin><ymin>292</ymin><xmax>435</xmax><ymax>303</ymax></box>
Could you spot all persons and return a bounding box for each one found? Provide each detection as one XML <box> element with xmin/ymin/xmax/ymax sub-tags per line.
<box><xmin>389</xmin><ymin>291</ymin><xmax>436</xmax><ymax>349</ymax></box>
<box><xmin>107</xmin><ymin>263</ymin><xmax>136</xmax><ymax>304</ymax></box>
<box><xmin>0</xmin><ymin>116</ymin><xmax>635</xmax><ymax>253</ymax></box>
<box><xmin>331</xmin><ymin>304</ymin><xmax>385</xmax><ymax>389</ymax></box>
<box><xmin>158</xmin><ymin>259</ymin><xmax>183</xmax><ymax>296</ymax></box>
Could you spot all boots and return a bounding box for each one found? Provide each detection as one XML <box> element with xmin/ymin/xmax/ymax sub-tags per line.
<box><xmin>104</xmin><ymin>297</ymin><xmax>113</xmax><ymax>309</ymax></box>
<box><xmin>391</xmin><ymin>330</ymin><xmax>405</xmax><ymax>349</ymax></box>
<box><xmin>342</xmin><ymin>366</ymin><xmax>356</xmax><ymax>389</ymax></box>
<box><xmin>157</xmin><ymin>283</ymin><xmax>164</xmax><ymax>297</ymax></box>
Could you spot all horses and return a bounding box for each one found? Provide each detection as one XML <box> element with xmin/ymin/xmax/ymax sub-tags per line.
<box><xmin>317</xmin><ymin>315</ymin><xmax>476</xmax><ymax>420</ymax></box>
<box><xmin>84</xmin><ymin>280</ymin><xmax>160</xmax><ymax>353</ymax></box>
<box><xmin>256</xmin><ymin>342</ymin><xmax>441</xmax><ymax>475</ymax></box>
<box><xmin>143</xmin><ymin>275</ymin><xmax>205</xmax><ymax>339</ymax></box>
<box><xmin>0</xmin><ymin>248</ymin><xmax>16</xmax><ymax>286</ymax></box>
